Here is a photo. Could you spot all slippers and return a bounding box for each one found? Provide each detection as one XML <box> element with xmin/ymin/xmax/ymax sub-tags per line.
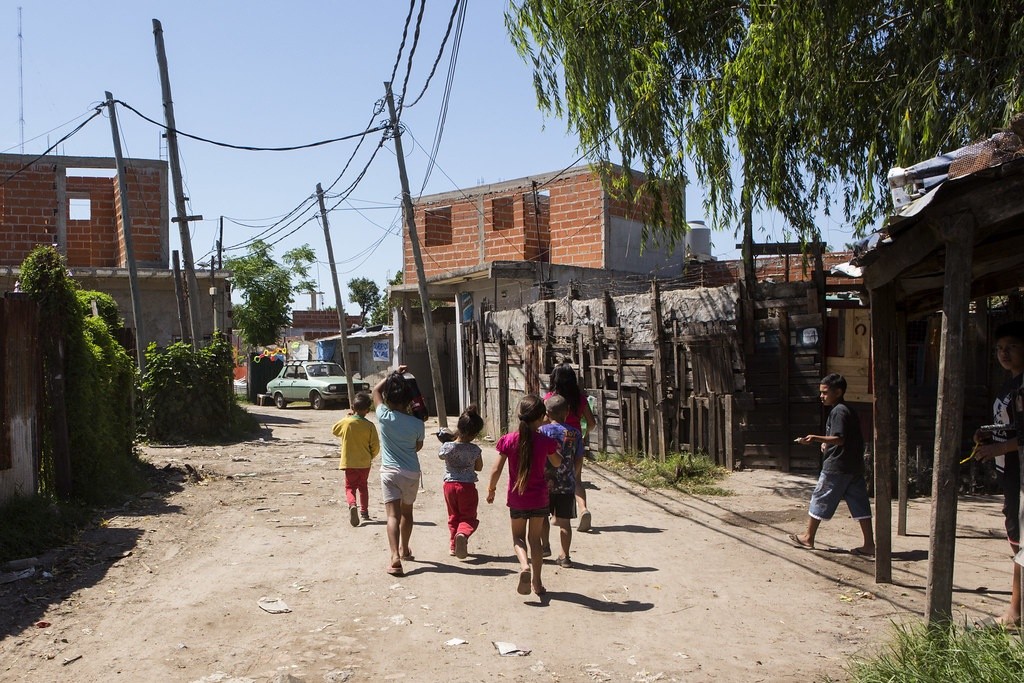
<box><xmin>788</xmin><ymin>534</ymin><xmax>815</xmax><ymax>550</ymax></box>
<box><xmin>387</xmin><ymin>563</ymin><xmax>403</xmax><ymax>574</ymax></box>
<box><xmin>400</xmin><ymin>546</ymin><xmax>412</xmax><ymax>560</ymax></box>
<box><xmin>974</xmin><ymin>617</ymin><xmax>1018</xmax><ymax>634</ymax></box>
<box><xmin>850</xmin><ymin>547</ymin><xmax>875</xmax><ymax>556</ymax></box>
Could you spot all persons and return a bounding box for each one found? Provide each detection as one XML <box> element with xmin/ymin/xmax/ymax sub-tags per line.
<box><xmin>438</xmin><ymin>404</ymin><xmax>485</xmax><ymax>558</ymax></box>
<box><xmin>788</xmin><ymin>374</ymin><xmax>876</xmax><ymax>561</ymax></box>
<box><xmin>536</xmin><ymin>394</ymin><xmax>583</xmax><ymax>567</ymax></box>
<box><xmin>332</xmin><ymin>393</ymin><xmax>380</xmax><ymax>526</ymax></box>
<box><xmin>543</xmin><ymin>363</ymin><xmax>596</xmax><ymax>532</ymax></box>
<box><xmin>971</xmin><ymin>330</ymin><xmax>1024</xmax><ymax>635</ymax></box>
<box><xmin>486</xmin><ymin>394</ymin><xmax>562</xmax><ymax>595</ymax></box>
<box><xmin>372</xmin><ymin>365</ymin><xmax>425</xmax><ymax>574</ymax></box>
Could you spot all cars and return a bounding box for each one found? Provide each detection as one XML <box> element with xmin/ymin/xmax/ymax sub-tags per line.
<box><xmin>266</xmin><ymin>360</ymin><xmax>371</xmax><ymax>410</ymax></box>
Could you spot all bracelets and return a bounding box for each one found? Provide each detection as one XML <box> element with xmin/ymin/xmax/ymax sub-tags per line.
<box><xmin>488</xmin><ymin>487</ymin><xmax>497</xmax><ymax>491</ymax></box>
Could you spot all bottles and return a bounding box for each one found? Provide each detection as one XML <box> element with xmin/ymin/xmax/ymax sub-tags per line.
<box><xmin>402</xmin><ymin>368</ymin><xmax>428</xmax><ymax>422</ymax></box>
<box><xmin>436</xmin><ymin>427</ymin><xmax>456</xmax><ymax>442</ymax></box>
<box><xmin>580</xmin><ymin>397</ymin><xmax>594</xmax><ymax>439</ymax></box>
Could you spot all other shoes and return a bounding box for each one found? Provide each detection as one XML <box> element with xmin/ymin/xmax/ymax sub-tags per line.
<box><xmin>517</xmin><ymin>570</ymin><xmax>532</xmax><ymax>596</ymax></box>
<box><xmin>542</xmin><ymin>545</ymin><xmax>552</xmax><ymax>557</ymax></box>
<box><xmin>350</xmin><ymin>506</ymin><xmax>359</xmax><ymax>526</ymax></box>
<box><xmin>450</xmin><ymin>551</ymin><xmax>455</xmax><ymax>555</ymax></box>
<box><xmin>577</xmin><ymin>511</ymin><xmax>591</xmax><ymax>532</ymax></box>
<box><xmin>455</xmin><ymin>534</ymin><xmax>468</xmax><ymax>559</ymax></box>
<box><xmin>361</xmin><ymin>514</ymin><xmax>369</xmax><ymax>520</ymax></box>
<box><xmin>556</xmin><ymin>556</ymin><xmax>573</xmax><ymax>568</ymax></box>
<box><xmin>534</xmin><ymin>588</ymin><xmax>546</xmax><ymax>594</ymax></box>
